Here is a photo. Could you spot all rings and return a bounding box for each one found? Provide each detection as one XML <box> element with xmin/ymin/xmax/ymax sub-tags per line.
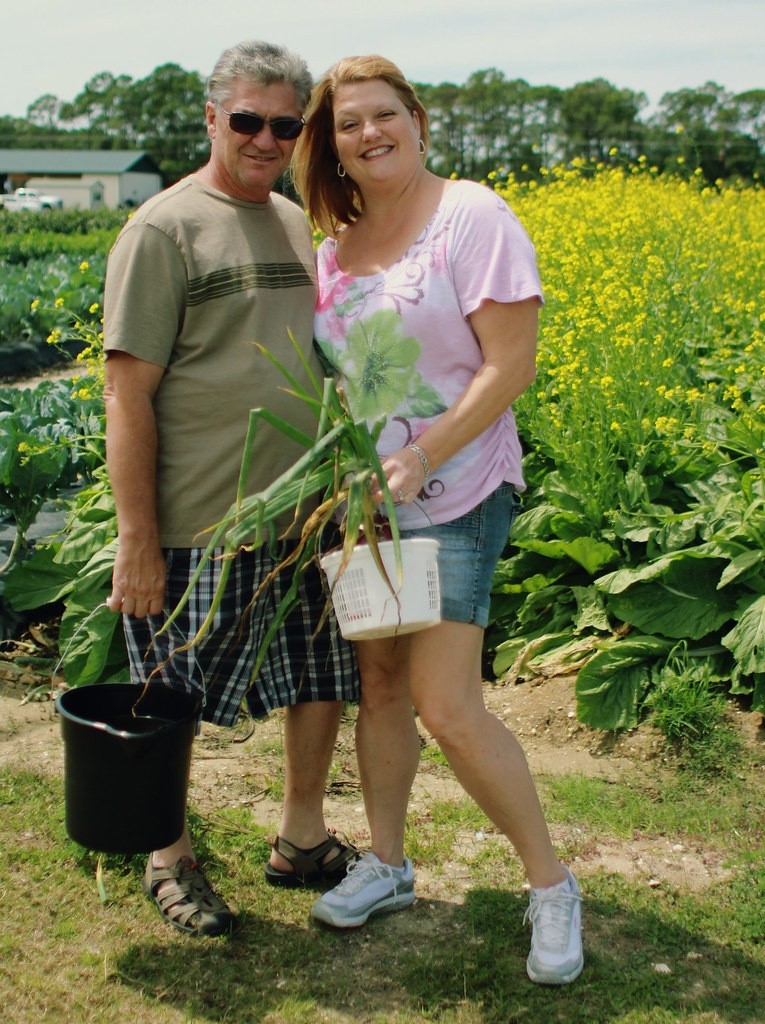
<box><xmin>397</xmin><ymin>490</ymin><xmax>405</xmax><ymax>502</ymax></box>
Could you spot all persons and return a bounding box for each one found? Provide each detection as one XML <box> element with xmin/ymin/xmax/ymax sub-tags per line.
<box><xmin>288</xmin><ymin>54</ymin><xmax>583</xmax><ymax>985</ymax></box>
<box><xmin>103</xmin><ymin>40</ymin><xmax>366</xmax><ymax>937</ymax></box>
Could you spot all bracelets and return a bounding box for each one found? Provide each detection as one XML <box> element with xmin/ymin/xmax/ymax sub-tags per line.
<box><xmin>405</xmin><ymin>444</ymin><xmax>429</xmax><ymax>479</ymax></box>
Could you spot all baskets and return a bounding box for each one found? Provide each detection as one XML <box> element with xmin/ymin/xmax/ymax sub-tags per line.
<box><xmin>319</xmin><ymin>538</ymin><xmax>440</xmax><ymax>642</ymax></box>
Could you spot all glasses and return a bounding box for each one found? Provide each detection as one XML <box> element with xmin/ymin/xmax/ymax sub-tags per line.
<box><xmin>213</xmin><ymin>100</ymin><xmax>307</xmax><ymax>141</ymax></box>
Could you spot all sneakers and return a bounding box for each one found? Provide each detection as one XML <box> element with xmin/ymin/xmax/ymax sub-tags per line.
<box><xmin>523</xmin><ymin>867</ymin><xmax>585</xmax><ymax>986</ymax></box>
<box><xmin>312</xmin><ymin>849</ymin><xmax>414</xmax><ymax>930</ymax></box>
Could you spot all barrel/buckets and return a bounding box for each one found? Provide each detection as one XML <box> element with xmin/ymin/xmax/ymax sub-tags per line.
<box><xmin>49</xmin><ymin>602</ymin><xmax>208</xmax><ymax>856</ymax></box>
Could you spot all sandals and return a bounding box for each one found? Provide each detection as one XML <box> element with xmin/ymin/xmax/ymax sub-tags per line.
<box><xmin>142</xmin><ymin>852</ymin><xmax>235</xmax><ymax>937</ymax></box>
<box><xmin>264</xmin><ymin>836</ymin><xmax>363</xmax><ymax>889</ymax></box>
